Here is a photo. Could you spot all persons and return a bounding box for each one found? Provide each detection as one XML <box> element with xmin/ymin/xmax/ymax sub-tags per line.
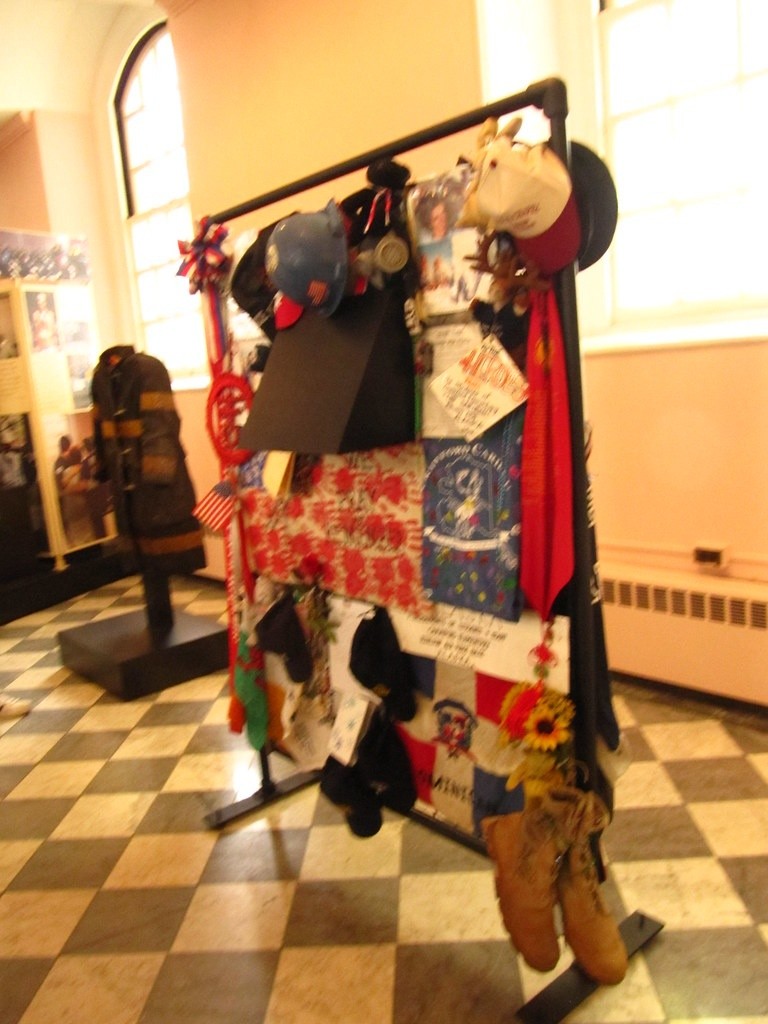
<box><xmin>31</xmin><ymin>295</ymin><xmax>58</xmax><ymax>349</ymax></box>
<box><xmin>54</xmin><ymin>434</ymin><xmax>108</xmax><ymax>548</ymax></box>
<box><xmin>417</xmin><ymin>195</ymin><xmax>448</xmax><ymax>238</ymax></box>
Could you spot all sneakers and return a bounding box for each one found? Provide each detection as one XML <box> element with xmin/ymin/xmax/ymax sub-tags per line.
<box><xmin>483</xmin><ymin>786</ymin><xmax>585</xmax><ymax>973</ymax></box>
<box><xmin>557</xmin><ymin>792</ymin><xmax>628</xmax><ymax>985</ymax></box>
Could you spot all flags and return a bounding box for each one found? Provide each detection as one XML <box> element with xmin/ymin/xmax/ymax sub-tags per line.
<box><xmin>193</xmin><ymin>482</ymin><xmax>238</xmax><ymax>533</ymax></box>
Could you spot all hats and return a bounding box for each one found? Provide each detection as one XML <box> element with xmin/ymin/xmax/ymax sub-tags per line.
<box><xmin>256</xmin><ymin>587</ymin><xmax>313</xmax><ymax>683</ymax></box>
<box><xmin>271</xmin><ymin>290</ymin><xmax>303</xmax><ymax>330</ymax></box>
<box><xmin>349</xmin><ymin>605</ymin><xmax>417</xmax><ymax>721</ymax></box>
<box><xmin>320</xmin><ymin>755</ymin><xmax>383</xmax><ymax>836</ymax></box>
<box><xmin>544</xmin><ymin>136</ymin><xmax>618</xmax><ymax>272</ymax></box>
<box><xmin>433</xmin><ymin>698</ymin><xmax>478</xmax><ymax>733</ymax></box>
<box><xmin>476</xmin><ymin>139</ymin><xmax>581</xmax><ymax>276</ymax></box>
<box><xmin>356</xmin><ymin>712</ymin><xmax>415</xmax><ymax>814</ymax></box>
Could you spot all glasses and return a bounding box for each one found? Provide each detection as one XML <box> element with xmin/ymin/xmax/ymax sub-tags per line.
<box><xmin>438</xmin><ymin>712</ymin><xmax>466</xmax><ymax>730</ymax></box>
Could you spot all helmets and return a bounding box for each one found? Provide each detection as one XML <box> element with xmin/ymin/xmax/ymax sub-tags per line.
<box><xmin>265</xmin><ymin>196</ymin><xmax>348</xmax><ymax>320</ymax></box>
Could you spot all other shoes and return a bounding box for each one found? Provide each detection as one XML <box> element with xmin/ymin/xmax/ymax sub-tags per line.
<box><xmin>0</xmin><ymin>693</ymin><xmax>33</xmax><ymax>718</ymax></box>
